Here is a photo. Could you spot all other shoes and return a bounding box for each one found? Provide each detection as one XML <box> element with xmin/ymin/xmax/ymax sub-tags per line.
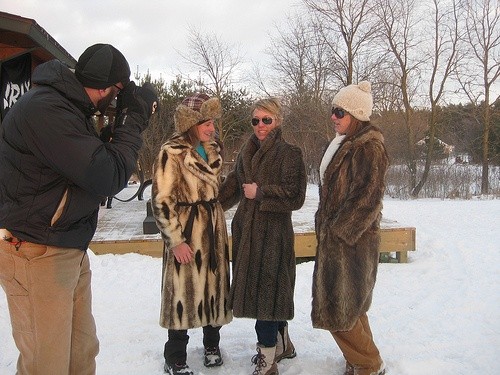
<box><xmin>107</xmin><ymin>202</ymin><xmax>112</xmax><ymax>208</ymax></box>
<box><xmin>101</xmin><ymin>201</ymin><xmax>105</xmax><ymax>206</ymax></box>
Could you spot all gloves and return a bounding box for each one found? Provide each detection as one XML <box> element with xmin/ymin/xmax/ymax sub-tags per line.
<box><xmin>116</xmin><ymin>81</ymin><xmax>161</xmax><ymax>132</ymax></box>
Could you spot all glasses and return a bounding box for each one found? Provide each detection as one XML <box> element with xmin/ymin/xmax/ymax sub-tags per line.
<box><xmin>251</xmin><ymin>115</ymin><xmax>273</xmax><ymax>126</ymax></box>
<box><xmin>331</xmin><ymin>106</ymin><xmax>346</xmax><ymax>119</ymax></box>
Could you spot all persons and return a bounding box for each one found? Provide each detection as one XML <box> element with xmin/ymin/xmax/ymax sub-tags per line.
<box><xmin>311</xmin><ymin>81</ymin><xmax>389</xmax><ymax>375</ymax></box>
<box><xmin>151</xmin><ymin>93</ymin><xmax>233</xmax><ymax>375</ymax></box>
<box><xmin>0</xmin><ymin>44</ymin><xmax>157</xmax><ymax>375</ymax></box>
<box><xmin>218</xmin><ymin>99</ymin><xmax>307</xmax><ymax>375</ymax></box>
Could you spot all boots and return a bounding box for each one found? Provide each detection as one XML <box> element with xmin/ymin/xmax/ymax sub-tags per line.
<box><xmin>250</xmin><ymin>342</ymin><xmax>280</xmax><ymax>375</ymax></box>
<box><xmin>273</xmin><ymin>322</ymin><xmax>297</xmax><ymax>363</ymax></box>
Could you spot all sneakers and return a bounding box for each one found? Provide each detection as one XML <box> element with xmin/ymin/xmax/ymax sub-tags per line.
<box><xmin>164</xmin><ymin>353</ymin><xmax>195</xmax><ymax>375</ymax></box>
<box><xmin>202</xmin><ymin>336</ymin><xmax>223</xmax><ymax>367</ymax></box>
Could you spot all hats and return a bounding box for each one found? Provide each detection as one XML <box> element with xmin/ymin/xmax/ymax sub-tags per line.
<box><xmin>332</xmin><ymin>81</ymin><xmax>374</xmax><ymax>121</ymax></box>
<box><xmin>75</xmin><ymin>43</ymin><xmax>132</xmax><ymax>90</ymax></box>
<box><xmin>173</xmin><ymin>93</ymin><xmax>223</xmax><ymax>133</ymax></box>
